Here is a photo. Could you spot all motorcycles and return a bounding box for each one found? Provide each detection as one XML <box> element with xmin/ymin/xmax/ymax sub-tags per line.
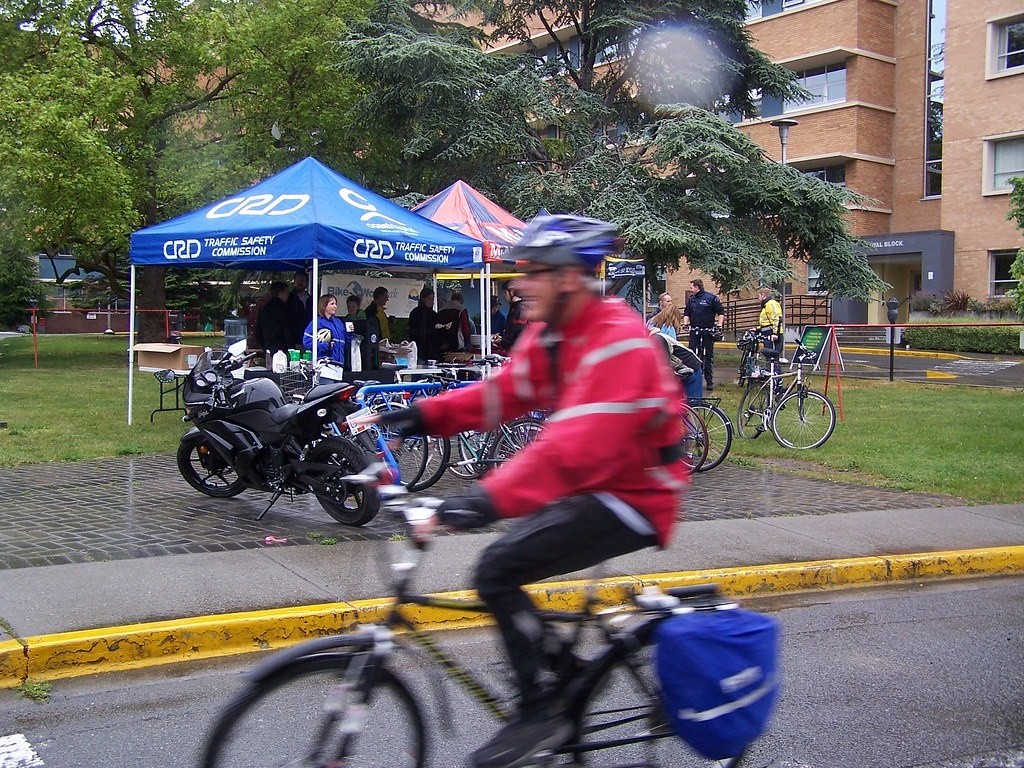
<box><xmin>153</xmin><ymin>337</ymin><xmax>382</xmax><ymax>528</ymax></box>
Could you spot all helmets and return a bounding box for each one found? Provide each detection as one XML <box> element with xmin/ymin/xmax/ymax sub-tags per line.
<box><xmin>485</xmin><ymin>295</ymin><xmax>501</xmax><ymax>308</ymax></box>
<box><xmin>736</xmin><ymin>339</ymin><xmax>753</xmax><ymax>350</ymax></box>
<box><xmin>498</xmin><ymin>214</ymin><xmax>624</xmax><ymax>274</ymax></box>
<box><xmin>317</xmin><ymin>329</ymin><xmax>332</xmax><ymax>343</ymax></box>
<box><xmin>502</xmin><ymin>280</ymin><xmax>520</xmax><ymax>289</ymax></box>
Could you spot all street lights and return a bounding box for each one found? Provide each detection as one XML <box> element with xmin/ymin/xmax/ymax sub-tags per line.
<box><xmin>770</xmin><ymin>118</ymin><xmax>800</xmax><ymax>166</ymax></box>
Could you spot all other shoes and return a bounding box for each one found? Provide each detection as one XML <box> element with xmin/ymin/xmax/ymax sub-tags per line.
<box><xmin>543</xmin><ymin>661</ymin><xmax>610</xmax><ymax>716</ymax></box>
<box><xmin>707</xmin><ymin>383</ymin><xmax>713</xmax><ymax>390</ymax></box>
<box><xmin>468</xmin><ymin>713</ymin><xmax>572</xmax><ymax>768</ymax></box>
<box><xmin>682</xmin><ymin>454</ymin><xmax>694</xmax><ymax>470</ymax></box>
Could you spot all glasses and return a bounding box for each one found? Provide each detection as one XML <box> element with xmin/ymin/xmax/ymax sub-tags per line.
<box><xmin>511</xmin><ymin>265</ymin><xmax>565</xmax><ymax>283</ymax></box>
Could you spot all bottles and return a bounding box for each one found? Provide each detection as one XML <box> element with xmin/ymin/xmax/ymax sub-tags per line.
<box><xmin>427</xmin><ymin>360</ymin><xmax>436</xmax><ymax>366</ymax></box>
<box><xmin>303</xmin><ymin>350</ymin><xmax>312</xmax><ymax>361</ymax></box>
<box><xmin>288</xmin><ymin>349</ymin><xmax>300</xmax><ymax>371</ymax></box>
<box><xmin>484</xmin><ymin>362</ymin><xmax>491</xmax><ymax>378</ymax></box>
<box><xmin>272</xmin><ymin>350</ymin><xmax>288</xmax><ymax>373</ymax></box>
<box><xmin>223</xmin><ymin>319</ymin><xmax>248</xmax><ymax>352</ymax></box>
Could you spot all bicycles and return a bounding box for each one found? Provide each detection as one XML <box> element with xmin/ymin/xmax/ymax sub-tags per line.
<box><xmin>236</xmin><ymin>353</ymin><xmax>737</xmax><ymax>493</ymax></box>
<box><xmin>736</xmin><ymin>338</ymin><xmax>837</xmax><ymax>452</ymax></box>
<box><xmin>193</xmin><ymin>418</ymin><xmax>761</xmax><ymax>768</ymax></box>
<box><xmin>736</xmin><ymin>330</ymin><xmax>776</xmax><ymax>392</ymax></box>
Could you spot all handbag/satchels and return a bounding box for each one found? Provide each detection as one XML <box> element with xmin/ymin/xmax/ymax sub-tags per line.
<box><xmin>658</xmin><ymin>609</ymin><xmax>779</xmax><ymax>761</ymax></box>
<box><xmin>378</xmin><ymin>338</ymin><xmax>418</xmax><ymax>370</ymax></box>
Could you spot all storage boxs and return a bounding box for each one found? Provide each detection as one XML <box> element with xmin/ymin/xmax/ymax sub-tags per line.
<box><xmin>394</xmin><ymin>375</ymin><xmax>412</xmax><ymax>382</ymax></box>
<box><xmin>223</xmin><ymin>319</ymin><xmax>248</xmax><ymax>335</ymax></box>
<box><xmin>225</xmin><ymin>335</ymin><xmax>248</xmax><ymax>348</ymax></box>
<box><xmin>126</xmin><ymin>342</ymin><xmax>204</xmax><ymax>370</ymax></box>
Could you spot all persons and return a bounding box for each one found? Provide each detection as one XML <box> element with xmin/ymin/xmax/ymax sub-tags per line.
<box><xmin>254</xmin><ymin>281</ymin><xmax>289</xmax><ymax>356</ymax></box>
<box><xmin>682</xmin><ymin>279</ymin><xmax>724</xmax><ymax>390</ymax></box>
<box><xmin>758</xmin><ymin>288</ymin><xmax>784</xmax><ymax>394</ymax></box>
<box><xmin>646</xmin><ymin>292</ymin><xmax>672</xmax><ymax>323</ymax></box>
<box><xmin>491</xmin><ymin>295</ymin><xmax>508</xmax><ymax>334</ymax></box>
<box><xmin>286</xmin><ymin>270</ymin><xmax>313</xmax><ymax>347</ymax></box>
<box><xmin>366</xmin><ymin>287</ymin><xmax>394</xmax><ymax>342</ymax></box>
<box><xmin>304</xmin><ymin>294</ymin><xmax>354</xmax><ymax>364</ymax></box>
<box><xmin>647</xmin><ymin>327</ymin><xmax>703</xmax><ymax>465</ymax></box>
<box><xmin>344</xmin><ymin>295</ymin><xmax>372</xmax><ymax>370</ymax></box>
<box><xmin>409</xmin><ymin>288</ymin><xmax>443</xmax><ymax>365</ymax></box>
<box><xmin>647</xmin><ymin>305</ymin><xmax>681</xmax><ymax>339</ymax></box>
<box><xmin>359</xmin><ymin>214</ymin><xmax>687</xmax><ymax>768</ymax></box>
<box><xmin>492</xmin><ymin>280</ymin><xmax>529</xmax><ymax>357</ymax></box>
<box><xmin>436</xmin><ymin>292</ymin><xmax>471</xmax><ymax>353</ymax></box>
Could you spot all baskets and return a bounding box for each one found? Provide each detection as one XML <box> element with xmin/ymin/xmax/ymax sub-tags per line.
<box><xmin>280</xmin><ymin>368</ymin><xmax>312</xmax><ymax>403</ymax></box>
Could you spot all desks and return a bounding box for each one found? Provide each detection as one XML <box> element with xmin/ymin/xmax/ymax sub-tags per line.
<box><xmin>243</xmin><ymin>369</ymin><xmax>314</xmax><ymax>399</ymax></box>
<box><xmin>138</xmin><ymin>366</ymin><xmax>192</xmax><ymax>423</ymax></box>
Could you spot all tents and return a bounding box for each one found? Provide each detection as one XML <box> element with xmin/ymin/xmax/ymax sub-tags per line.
<box><xmin>528</xmin><ymin>208</ymin><xmax>646</xmax><ymax>327</ymax></box>
<box><xmin>410</xmin><ymin>180</ymin><xmax>530</xmax><ymax>378</ymax></box>
<box><xmin>128</xmin><ymin>157</ymin><xmax>485</xmax><ymax>426</ymax></box>
